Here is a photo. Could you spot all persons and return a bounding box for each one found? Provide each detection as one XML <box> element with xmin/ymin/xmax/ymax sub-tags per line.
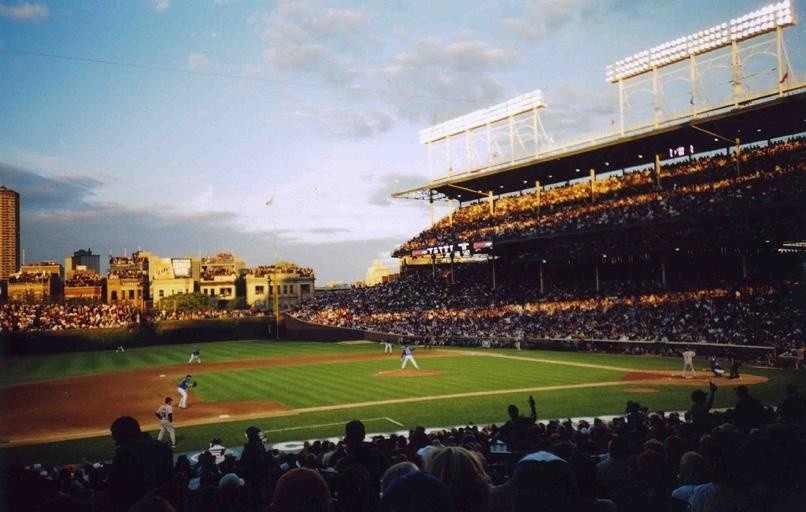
<box><xmin>756</xmin><ymin>356</ymin><xmax>765</xmax><ymax>365</ymax></box>
<box><xmin>116</xmin><ymin>342</ymin><xmax>124</xmax><ymax>352</ymax></box>
<box><xmin>188</xmin><ymin>347</ymin><xmax>201</xmax><ymax>365</ymax></box>
<box><xmin>1</xmin><ymin>253</ymin><xmax>285</xmax><ymax>333</ymax></box>
<box><xmin>400</xmin><ymin>345</ymin><xmax>421</xmax><ymax>370</ymax></box>
<box><xmin>285</xmin><ymin>132</ymin><xmax>806</xmax><ymax>359</ymax></box>
<box><xmin>765</xmin><ymin>346</ymin><xmax>806</xmax><ymax>369</ymax></box>
<box><xmin>681</xmin><ymin>347</ymin><xmax>741</xmax><ymax>380</ymax></box>
<box><xmin>1</xmin><ymin>383</ymin><xmax>805</xmax><ymax>511</ymax></box>
<box><xmin>176</xmin><ymin>374</ymin><xmax>199</xmax><ymax>408</ymax></box>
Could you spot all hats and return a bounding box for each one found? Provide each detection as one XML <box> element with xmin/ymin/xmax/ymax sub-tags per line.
<box><xmin>166</xmin><ymin>397</ymin><xmax>173</xmax><ymax>403</ymax></box>
<box><xmin>219</xmin><ymin>473</ymin><xmax>245</xmax><ymax>489</ymax></box>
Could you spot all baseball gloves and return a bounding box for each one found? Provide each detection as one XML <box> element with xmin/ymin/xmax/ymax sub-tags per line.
<box><xmin>192</xmin><ymin>382</ymin><xmax>197</xmax><ymax>387</ymax></box>
